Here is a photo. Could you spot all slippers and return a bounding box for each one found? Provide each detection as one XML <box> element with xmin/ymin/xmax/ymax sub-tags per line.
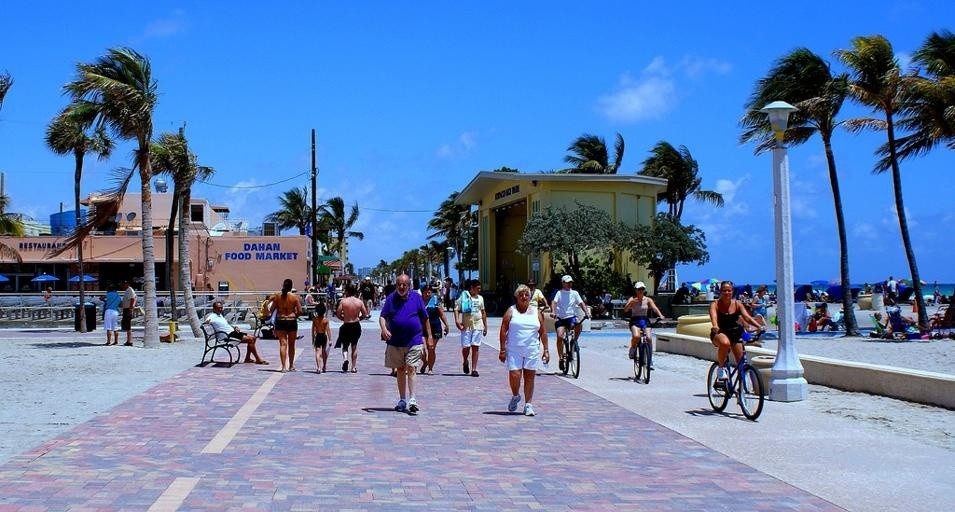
<box><xmin>256</xmin><ymin>361</ymin><xmax>269</xmax><ymax>365</ymax></box>
<box><xmin>245</xmin><ymin>358</ymin><xmax>255</xmax><ymax>363</ymax></box>
<box><xmin>342</xmin><ymin>360</ymin><xmax>348</xmax><ymax>372</ymax></box>
<box><xmin>351</xmin><ymin>368</ymin><xmax>357</xmax><ymax>373</ymax></box>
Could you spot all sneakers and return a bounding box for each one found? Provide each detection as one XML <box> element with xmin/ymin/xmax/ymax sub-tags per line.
<box><xmin>408</xmin><ymin>400</ymin><xmax>419</xmax><ymax>412</ymax></box>
<box><xmin>428</xmin><ymin>370</ymin><xmax>434</xmax><ymax>375</ymax></box>
<box><xmin>649</xmin><ymin>363</ymin><xmax>654</xmax><ymax>370</ymax></box>
<box><xmin>394</xmin><ymin>399</ymin><xmax>406</xmax><ymax>412</ymax></box>
<box><xmin>462</xmin><ymin>360</ymin><xmax>470</xmax><ymax>374</ymax></box>
<box><xmin>629</xmin><ymin>347</ymin><xmax>635</xmax><ymax>360</ymax></box>
<box><xmin>124</xmin><ymin>342</ymin><xmax>133</xmax><ymax>346</ymax></box>
<box><xmin>523</xmin><ymin>403</ymin><xmax>535</xmax><ymax>416</ymax></box>
<box><xmin>508</xmin><ymin>394</ymin><xmax>521</xmax><ymax>411</ymax></box>
<box><xmin>559</xmin><ymin>359</ymin><xmax>565</xmax><ymax>370</ymax></box>
<box><xmin>717</xmin><ymin>368</ymin><xmax>728</xmax><ymax>381</ymax></box>
<box><xmin>471</xmin><ymin>370</ymin><xmax>479</xmax><ymax>377</ymax></box>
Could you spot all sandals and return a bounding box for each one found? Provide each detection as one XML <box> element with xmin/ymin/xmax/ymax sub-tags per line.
<box><xmin>282</xmin><ymin>368</ymin><xmax>286</xmax><ymax>373</ymax></box>
<box><xmin>317</xmin><ymin>369</ymin><xmax>321</xmax><ymax>374</ymax></box>
<box><xmin>289</xmin><ymin>364</ymin><xmax>296</xmax><ymax>372</ymax></box>
<box><xmin>322</xmin><ymin>368</ymin><xmax>326</xmax><ymax>372</ymax></box>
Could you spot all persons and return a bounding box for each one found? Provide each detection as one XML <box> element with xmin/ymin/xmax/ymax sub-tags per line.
<box><xmin>334</xmin><ymin>283</ymin><xmax>369</xmax><ymax>373</ymax></box>
<box><xmin>379</xmin><ymin>274</ymin><xmax>435</xmax><ymax>416</ymax></box>
<box><xmin>498</xmin><ymin>283</ymin><xmax>552</xmax><ymax>417</ymax></box>
<box><xmin>265</xmin><ymin>278</ymin><xmax>304</xmax><ymax>373</ymax></box>
<box><xmin>118</xmin><ymin>279</ymin><xmax>137</xmax><ymax>346</ymax></box>
<box><xmin>258</xmin><ymin>294</ymin><xmax>303</xmax><ymax>339</ymax></box>
<box><xmin>420</xmin><ymin>285</ymin><xmax>449</xmax><ymax>373</ymax></box>
<box><xmin>310</xmin><ymin>302</ymin><xmax>333</xmax><ymax>375</ymax></box>
<box><xmin>550</xmin><ymin>275</ymin><xmax>592</xmax><ymax>369</ymax></box>
<box><xmin>99</xmin><ymin>283</ymin><xmax>121</xmax><ymax>346</ymax></box>
<box><xmin>527</xmin><ymin>279</ymin><xmax>549</xmax><ymax>309</ymax></box>
<box><xmin>191</xmin><ymin>282</ymin><xmax>196</xmax><ymax>299</ymax></box>
<box><xmin>206</xmin><ymin>283</ymin><xmax>214</xmax><ymax>301</ymax></box>
<box><xmin>453</xmin><ymin>280</ymin><xmax>488</xmax><ymax>377</ymax></box>
<box><xmin>668</xmin><ymin>277</ymin><xmax>955</xmax><ymax>339</ymax></box>
<box><xmin>43</xmin><ymin>286</ymin><xmax>53</xmax><ymax>306</ymax></box>
<box><xmin>709</xmin><ymin>280</ymin><xmax>769</xmax><ymax>406</ymax></box>
<box><xmin>592</xmin><ymin>288</ymin><xmax>614</xmax><ymax>319</ymax></box>
<box><xmin>297</xmin><ymin>275</ymin><xmax>459</xmax><ymax>322</ymax></box>
<box><xmin>622</xmin><ymin>280</ymin><xmax>665</xmax><ymax>372</ymax></box>
<box><xmin>203</xmin><ymin>300</ymin><xmax>269</xmax><ymax>365</ymax></box>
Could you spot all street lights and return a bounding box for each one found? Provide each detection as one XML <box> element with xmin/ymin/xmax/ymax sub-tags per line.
<box><xmin>759</xmin><ymin>100</ymin><xmax>815</xmax><ymax>402</ymax></box>
<box><xmin>371</xmin><ymin>245</ymin><xmax>462</xmax><ymax>291</ymax></box>
<box><xmin>153</xmin><ymin>170</ymin><xmax>181</xmax><ymax>323</ymax></box>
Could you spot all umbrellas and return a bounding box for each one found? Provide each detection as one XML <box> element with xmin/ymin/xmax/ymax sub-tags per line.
<box><xmin>29</xmin><ymin>272</ymin><xmax>61</xmax><ymax>290</ymax></box>
<box><xmin>66</xmin><ymin>273</ymin><xmax>98</xmax><ymax>284</ymax></box>
<box><xmin>0</xmin><ymin>274</ymin><xmax>10</xmax><ymax>283</ymax></box>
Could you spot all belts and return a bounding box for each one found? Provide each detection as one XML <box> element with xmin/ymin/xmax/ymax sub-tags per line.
<box><xmin>276</xmin><ymin>317</ymin><xmax>297</xmax><ymax>321</ymax></box>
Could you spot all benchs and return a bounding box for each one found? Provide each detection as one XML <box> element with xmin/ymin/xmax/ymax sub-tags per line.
<box><xmin>611</xmin><ymin>299</ymin><xmax>633</xmax><ymax>320</ymax></box>
<box><xmin>200</xmin><ymin>322</ymin><xmax>242</xmax><ymax>368</ymax></box>
<box><xmin>0</xmin><ymin>293</ymin><xmax>248</xmax><ymax>324</ymax></box>
<box><xmin>252</xmin><ymin>311</ymin><xmax>272</xmax><ymax>338</ymax></box>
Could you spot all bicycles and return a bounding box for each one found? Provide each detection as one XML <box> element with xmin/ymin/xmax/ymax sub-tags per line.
<box><xmin>705</xmin><ymin>326</ymin><xmax>766</xmax><ymax>422</ymax></box>
<box><xmin>548</xmin><ymin>313</ymin><xmax>587</xmax><ymax>381</ymax></box>
<box><xmin>620</xmin><ymin>317</ymin><xmax>661</xmax><ymax>386</ymax></box>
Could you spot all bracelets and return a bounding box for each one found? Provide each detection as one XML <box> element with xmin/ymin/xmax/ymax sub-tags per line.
<box><xmin>500</xmin><ymin>350</ymin><xmax>506</xmax><ymax>354</ymax></box>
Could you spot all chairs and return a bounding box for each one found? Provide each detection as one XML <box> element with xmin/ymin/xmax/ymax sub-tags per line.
<box><xmin>826</xmin><ymin>299</ymin><xmax>858</xmax><ymax>332</ymax></box>
<box><xmin>868</xmin><ymin>313</ymin><xmax>891</xmax><ymax>334</ymax></box>
<box><xmin>886</xmin><ymin>304</ymin><xmax>922</xmax><ymax>339</ymax></box>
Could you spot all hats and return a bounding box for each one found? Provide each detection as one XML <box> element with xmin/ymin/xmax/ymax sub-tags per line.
<box><xmin>561</xmin><ymin>275</ymin><xmax>574</xmax><ymax>283</ymax></box>
<box><xmin>364</xmin><ymin>277</ymin><xmax>371</xmax><ymax>280</ymax></box>
<box><xmin>527</xmin><ymin>279</ymin><xmax>536</xmax><ymax>285</ymax></box>
<box><xmin>635</xmin><ymin>282</ymin><xmax>646</xmax><ymax>289</ymax></box>
